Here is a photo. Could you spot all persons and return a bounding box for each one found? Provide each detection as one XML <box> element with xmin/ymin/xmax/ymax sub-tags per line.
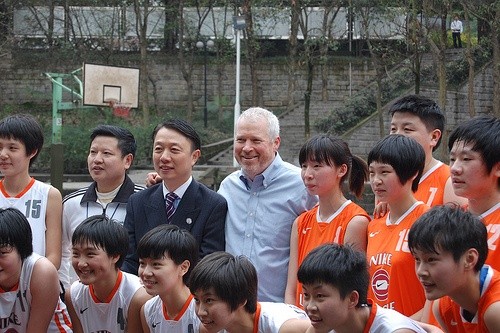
<box><xmin>145</xmin><ymin>108</ymin><xmax>319</xmax><ymax>304</ymax></box>
<box><xmin>1</xmin><ymin>94</ymin><xmax>500</xmax><ymax>333</ymax></box>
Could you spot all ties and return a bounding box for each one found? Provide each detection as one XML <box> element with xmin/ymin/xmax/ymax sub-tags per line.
<box><xmin>165</xmin><ymin>193</ymin><xmax>180</xmax><ymax>224</ymax></box>
